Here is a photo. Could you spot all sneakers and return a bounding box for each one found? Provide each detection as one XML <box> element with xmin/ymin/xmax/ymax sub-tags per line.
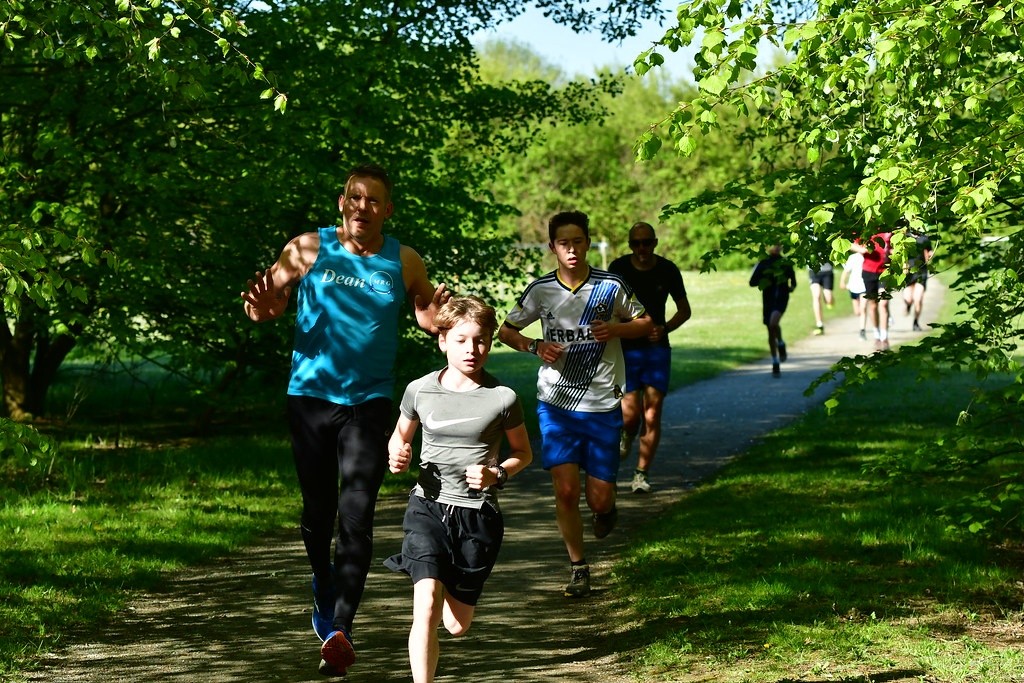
<box><xmin>826</xmin><ymin>303</ymin><xmax>833</xmax><ymax>311</ymax></box>
<box><xmin>592</xmin><ymin>506</ymin><xmax>618</xmax><ymax>539</ymax></box>
<box><xmin>632</xmin><ymin>468</ymin><xmax>651</xmax><ymax>494</ymax></box>
<box><xmin>813</xmin><ymin>326</ymin><xmax>824</xmax><ymax>337</ymax></box>
<box><xmin>565</xmin><ymin>565</ymin><xmax>591</xmax><ymax>598</ymax></box>
<box><xmin>312</xmin><ymin>562</ymin><xmax>341</xmax><ymax>642</ymax></box>
<box><xmin>318</xmin><ymin>625</ymin><xmax>357</xmax><ymax>678</ymax></box>
<box><xmin>619</xmin><ymin>429</ymin><xmax>634</xmax><ymax>457</ymax></box>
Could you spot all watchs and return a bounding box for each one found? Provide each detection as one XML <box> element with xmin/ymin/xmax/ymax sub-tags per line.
<box><xmin>530</xmin><ymin>339</ymin><xmax>544</xmax><ymax>355</ymax></box>
<box><xmin>489</xmin><ymin>464</ymin><xmax>507</xmax><ymax>490</ymax></box>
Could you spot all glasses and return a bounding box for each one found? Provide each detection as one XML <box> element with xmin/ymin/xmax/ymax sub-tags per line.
<box><xmin>628</xmin><ymin>238</ymin><xmax>654</xmax><ymax>248</ymax></box>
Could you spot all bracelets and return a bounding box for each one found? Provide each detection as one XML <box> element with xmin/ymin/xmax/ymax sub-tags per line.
<box><xmin>661</xmin><ymin>324</ymin><xmax>668</xmax><ymax>334</ymax></box>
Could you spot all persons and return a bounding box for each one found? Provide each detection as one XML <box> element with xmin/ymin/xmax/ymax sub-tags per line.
<box><xmin>808</xmin><ymin>262</ymin><xmax>833</xmax><ymax>335</ymax></box>
<box><xmin>498</xmin><ymin>210</ymin><xmax>654</xmax><ymax>597</ymax></box>
<box><xmin>241</xmin><ymin>163</ymin><xmax>455</xmax><ymax>674</ymax></box>
<box><xmin>901</xmin><ymin>237</ymin><xmax>933</xmax><ymax>331</ymax></box>
<box><xmin>608</xmin><ymin>221</ymin><xmax>691</xmax><ymax>494</ymax></box>
<box><xmin>749</xmin><ymin>244</ymin><xmax>797</xmax><ymax>378</ymax></box>
<box><xmin>383</xmin><ymin>294</ymin><xmax>533</xmax><ymax>682</ymax></box>
<box><xmin>850</xmin><ymin>232</ymin><xmax>893</xmax><ymax>348</ymax></box>
<box><xmin>840</xmin><ymin>238</ymin><xmax>869</xmax><ymax>342</ymax></box>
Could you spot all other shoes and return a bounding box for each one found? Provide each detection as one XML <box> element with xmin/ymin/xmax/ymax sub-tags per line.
<box><xmin>904</xmin><ymin>304</ymin><xmax>911</xmax><ymax>317</ymax></box>
<box><xmin>772</xmin><ymin>362</ymin><xmax>781</xmax><ymax>378</ymax></box>
<box><xmin>859</xmin><ymin>329</ymin><xmax>866</xmax><ymax>339</ymax></box>
<box><xmin>913</xmin><ymin>319</ymin><xmax>922</xmax><ymax>332</ymax></box>
<box><xmin>882</xmin><ymin>339</ymin><xmax>890</xmax><ymax>353</ymax></box>
<box><xmin>873</xmin><ymin>338</ymin><xmax>881</xmax><ymax>350</ymax></box>
<box><xmin>779</xmin><ymin>341</ymin><xmax>787</xmax><ymax>362</ymax></box>
